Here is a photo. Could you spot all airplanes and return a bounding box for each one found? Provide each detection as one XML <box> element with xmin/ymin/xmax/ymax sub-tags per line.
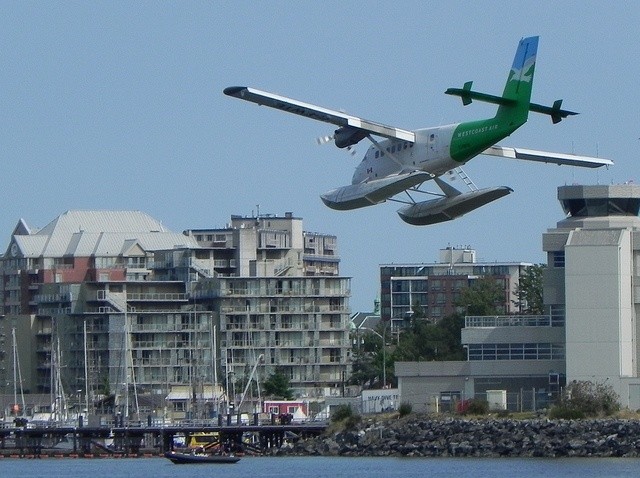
<box><xmin>220</xmin><ymin>34</ymin><xmax>616</xmax><ymax>227</ymax></box>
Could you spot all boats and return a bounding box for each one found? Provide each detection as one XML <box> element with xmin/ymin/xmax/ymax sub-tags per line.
<box><xmin>164</xmin><ymin>438</ymin><xmax>241</xmax><ymax>464</ymax></box>
